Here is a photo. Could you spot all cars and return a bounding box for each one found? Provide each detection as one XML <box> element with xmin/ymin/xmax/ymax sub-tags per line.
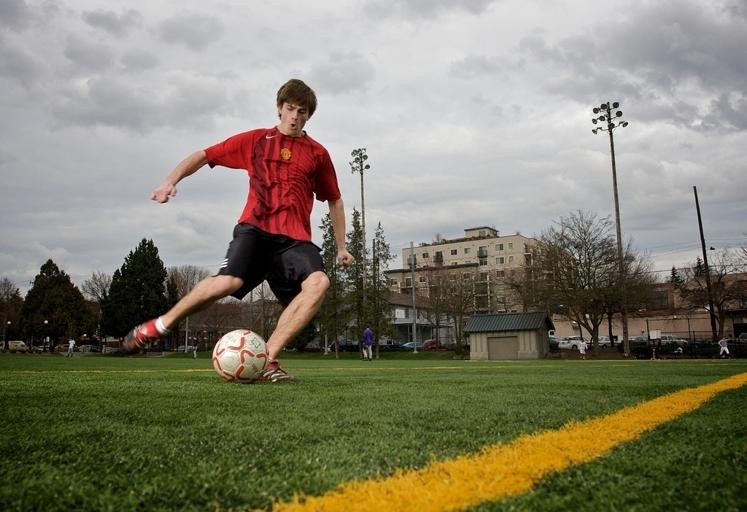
<box><xmin>0</xmin><ymin>335</ymin><xmax>208</xmax><ymax>354</ymax></box>
<box><xmin>549</xmin><ymin>332</ymin><xmax>747</xmax><ymax>356</ymax></box>
<box><xmin>300</xmin><ymin>334</ymin><xmax>470</xmax><ymax>352</ymax></box>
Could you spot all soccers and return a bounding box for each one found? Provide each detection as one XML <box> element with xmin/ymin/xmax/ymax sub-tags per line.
<box><xmin>213</xmin><ymin>329</ymin><xmax>267</xmax><ymax>381</ymax></box>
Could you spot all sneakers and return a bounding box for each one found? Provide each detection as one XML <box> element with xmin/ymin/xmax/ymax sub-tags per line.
<box><xmin>258</xmin><ymin>357</ymin><xmax>298</xmax><ymax>383</ymax></box>
<box><xmin>123</xmin><ymin>320</ymin><xmax>164</xmax><ymax>352</ymax></box>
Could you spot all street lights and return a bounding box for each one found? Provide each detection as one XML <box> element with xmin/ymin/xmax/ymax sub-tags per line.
<box><xmin>42</xmin><ymin>319</ymin><xmax>49</xmax><ymax>352</ymax></box>
<box><xmin>348</xmin><ymin>147</ymin><xmax>371</xmax><ymax>330</ymax></box>
<box><xmin>6</xmin><ymin>320</ymin><xmax>11</xmax><ymax>339</ymax></box>
<box><xmin>589</xmin><ymin>100</ymin><xmax>632</xmax><ymax>357</ymax></box>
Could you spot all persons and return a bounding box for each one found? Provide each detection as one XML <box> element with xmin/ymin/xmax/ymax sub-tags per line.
<box><xmin>119</xmin><ymin>78</ymin><xmax>354</xmax><ymax>385</ymax></box>
<box><xmin>576</xmin><ymin>338</ymin><xmax>587</xmax><ymax>360</ymax></box>
<box><xmin>361</xmin><ymin>324</ymin><xmax>374</xmax><ymax>361</ymax></box>
<box><xmin>716</xmin><ymin>336</ymin><xmax>731</xmax><ymax>360</ymax></box>
<box><xmin>65</xmin><ymin>337</ymin><xmax>75</xmax><ymax>358</ymax></box>
